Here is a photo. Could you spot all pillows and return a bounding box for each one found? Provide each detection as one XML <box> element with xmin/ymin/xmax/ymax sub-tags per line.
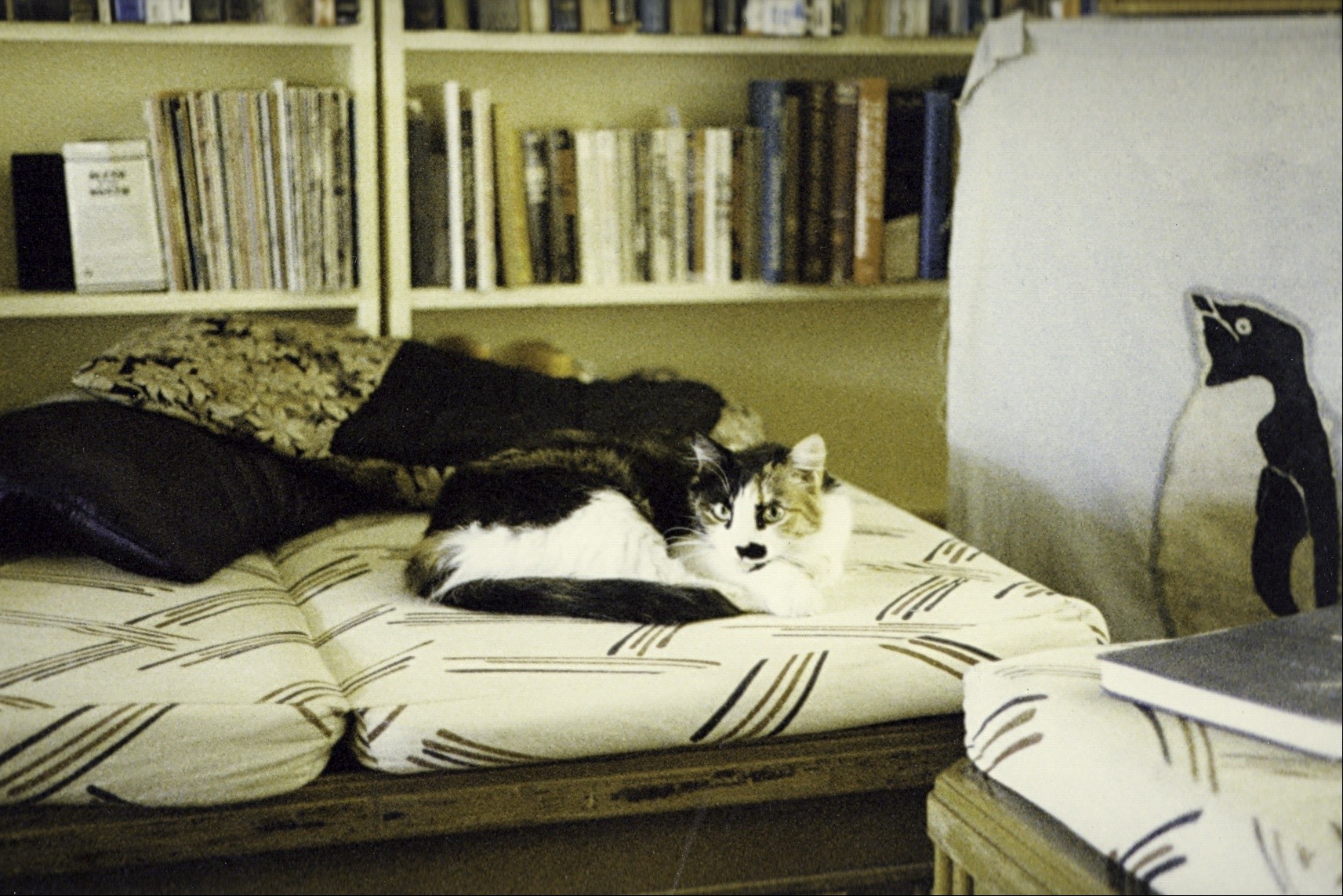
<box><xmin>73</xmin><ymin>313</ymin><xmax>455</xmax><ymax>500</ymax></box>
<box><xmin>0</xmin><ymin>402</ymin><xmax>346</xmax><ymax>581</ymax></box>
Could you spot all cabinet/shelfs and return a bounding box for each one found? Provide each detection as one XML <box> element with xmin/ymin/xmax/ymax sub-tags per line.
<box><xmin>0</xmin><ymin>0</ymin><xmax>379</xmax><ymax>335</ymax></box>
<box><xmin>380</xmin><ymin>1</ymin><xmax>1083</xmax><ymax>308</ymax></box>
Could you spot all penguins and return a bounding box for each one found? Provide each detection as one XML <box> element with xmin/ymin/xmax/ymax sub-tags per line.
<box><xmin>1153</xmin><ymin>286</ymin><xmax>1343</xmax><ymax>638</ymax></box>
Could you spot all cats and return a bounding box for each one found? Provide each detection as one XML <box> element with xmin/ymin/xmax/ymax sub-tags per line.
<box><xmin>407</xmin><ymin>431</ymin><xmax>853</xmax><ymax>622</ymax></box>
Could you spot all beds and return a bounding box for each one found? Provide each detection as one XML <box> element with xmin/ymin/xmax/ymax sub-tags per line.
<box><xmin>0</xmin><ymin>445</ymin><xmax>1116</xmax><ymax>895</ymax></box>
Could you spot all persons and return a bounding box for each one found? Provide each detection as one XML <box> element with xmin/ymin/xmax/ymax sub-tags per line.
<box><xmin>0</xmin><ymin>0</ymin><xmax>1094</xmax><ymax>294</ymax></box>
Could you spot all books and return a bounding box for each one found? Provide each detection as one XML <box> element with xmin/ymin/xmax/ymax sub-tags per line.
<box><xmin>1094</xmin><ymin>602</ymin><xmax>1343</xmax><ymax>764</ymax></box>
